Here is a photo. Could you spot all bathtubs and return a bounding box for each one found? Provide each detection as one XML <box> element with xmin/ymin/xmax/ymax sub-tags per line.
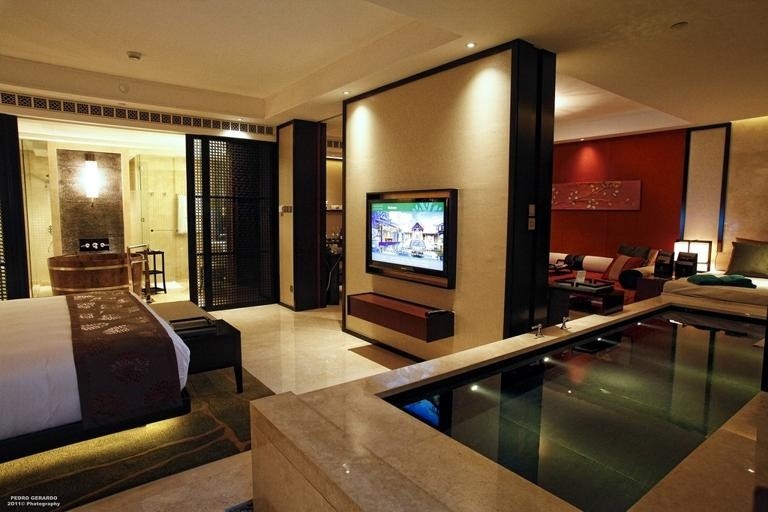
<box><xmin>48</xmin><ymin>253</ymin><xmax>142</xmax><ymax>298</ymax></box>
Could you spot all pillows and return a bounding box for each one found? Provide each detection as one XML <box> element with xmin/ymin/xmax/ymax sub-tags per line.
<box><xmin>601</xmin><ymin>244</ymin><xmax>660</xmax><ymax>281</ymax></box>
<box><xmin>728</xmin><ymin>238</ymin><xmax>768</xmax><ymax>278</ymax></box>
<box><xmin>724</xmin><ymin>242</ymin><xmax>768</xmax><ymax>277</ymax></box>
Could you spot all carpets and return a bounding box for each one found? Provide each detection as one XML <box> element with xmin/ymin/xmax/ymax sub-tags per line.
<box><xmin>0</xmin><ymin>366</ymin><xmax>275</xmax><ymax>511</ymax></box>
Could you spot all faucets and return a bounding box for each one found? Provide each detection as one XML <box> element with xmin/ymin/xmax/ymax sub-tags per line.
<box><xmin>92</xmin><ymin>243</ymin><xmax>98</xmax><ymax>249</ymax></box>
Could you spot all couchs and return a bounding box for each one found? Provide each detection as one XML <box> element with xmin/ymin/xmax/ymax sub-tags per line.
<box><xmin>548</xmin><ymin>252</ymin><xmax>654</xmax><ymax>305</ymax></box>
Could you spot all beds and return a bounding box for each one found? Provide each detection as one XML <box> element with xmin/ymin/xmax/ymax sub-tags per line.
<box><xmin>0</xmin><ymin>287</ymin><xmax>192</xmax><ymax>453</ymax></box>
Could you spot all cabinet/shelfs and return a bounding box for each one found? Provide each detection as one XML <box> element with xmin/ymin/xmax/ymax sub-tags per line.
<box><xmin>136</xmin><ymin>251</ymin><xmax>167</xmax><ymax>295</ymax></box>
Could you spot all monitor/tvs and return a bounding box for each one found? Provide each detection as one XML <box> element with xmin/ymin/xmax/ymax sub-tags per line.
<box><xmin>404</xmin><ymin>391</ymin><xmax>453</xmax><ymax>436</ymax></box>
<box><xmin>366</xmin><ymin>189</ymin><xmax>458</xmax><ymax>289</ymax></box>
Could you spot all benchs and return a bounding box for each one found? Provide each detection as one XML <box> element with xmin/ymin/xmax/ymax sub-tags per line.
<box><xmin>149</xmin><ymin>301</ymin><xmax>243</xmax><ymax>394</ymax></box>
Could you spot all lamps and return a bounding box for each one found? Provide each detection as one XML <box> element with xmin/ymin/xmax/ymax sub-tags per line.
<box><xmin>674</xmin><ymin>241</ymin><xmax>712</xmax><ymax>273</ymax></box>
<box><xmin>84</xmin><ymin>154</ymin><xmax>99</xmax><ymax>208</ymax></box>
